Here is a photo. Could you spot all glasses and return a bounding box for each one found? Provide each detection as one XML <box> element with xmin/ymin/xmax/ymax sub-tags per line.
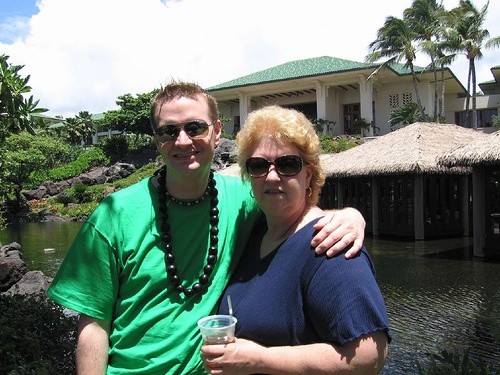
<box><xmin>153</xmin><ymin>120</ymin><xmax>215</xmax><ymax>141</ymax></box>
<box><xmin>245</xmin><ymin>154</ymin><xmax>307</xmax><ymax>178</ymax></box>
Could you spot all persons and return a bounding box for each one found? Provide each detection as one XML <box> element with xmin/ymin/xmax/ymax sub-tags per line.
<box><xmin>199</xmin><ymin>105</ymin><xmax>392</xmax><ymax>375</ymax></box>
<box><xmin>46</xmin><ymin>77</ymin><xmax>367</xmax><ymax>375</ymax></box>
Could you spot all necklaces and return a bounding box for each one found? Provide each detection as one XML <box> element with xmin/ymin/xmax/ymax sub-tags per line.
<box><xmin>157</xmin><ymin>166</ymin><xmax>219</xmax><ymax>297</ymax></box>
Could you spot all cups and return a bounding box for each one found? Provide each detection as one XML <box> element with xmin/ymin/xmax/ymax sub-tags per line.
<box><xmin>197</xmin><ymin>314</ymin><xmax>238</xmax><ymax>370</ymax></box>
